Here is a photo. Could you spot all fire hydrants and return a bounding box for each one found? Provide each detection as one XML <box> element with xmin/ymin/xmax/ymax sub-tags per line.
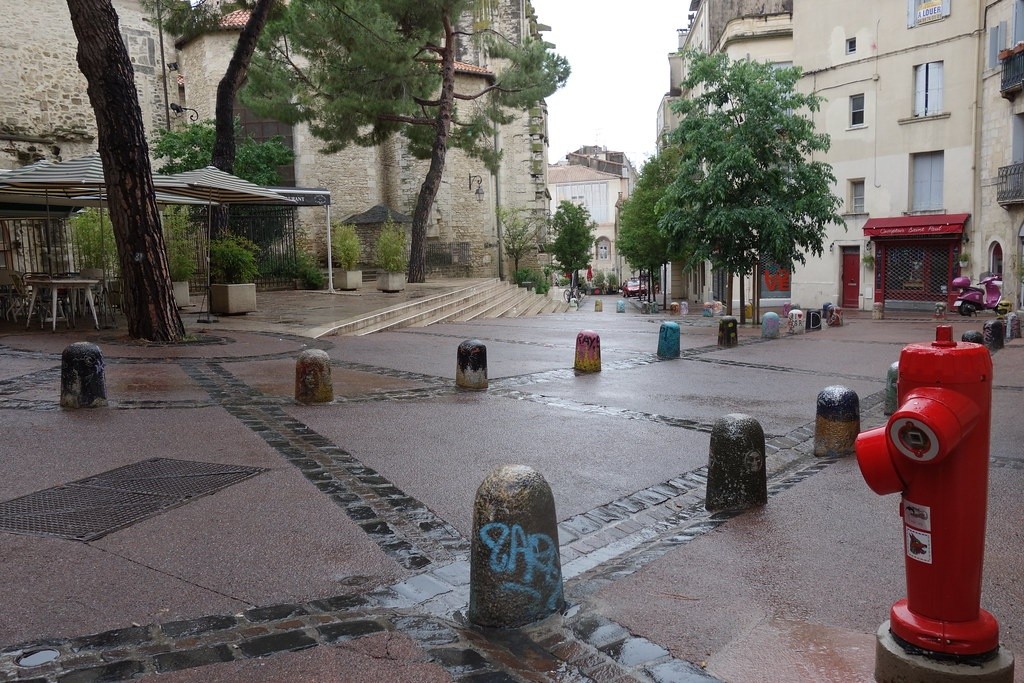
<box><xmin>854</xmin><ymin>324</ymin><xmax>1004</xmax><ymax>665</ymax></box>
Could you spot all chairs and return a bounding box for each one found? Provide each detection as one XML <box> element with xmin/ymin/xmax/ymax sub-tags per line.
<box><xmin>0</xmin><ymin>273</ymin><xmax>128</xmax><ymax>330</ymax></box>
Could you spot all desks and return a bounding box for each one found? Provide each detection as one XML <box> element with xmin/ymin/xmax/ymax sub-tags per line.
<box><xmin>25</xmin><ymin>279</ymin><xmax>100</xmax><ymax>334</ymax></box>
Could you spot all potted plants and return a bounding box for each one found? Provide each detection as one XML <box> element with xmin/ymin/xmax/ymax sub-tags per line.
<box><xmin>958</xmin><ymin>252</ymin><xmax>969</xmax><ymax>267</ymax></box>
<box><xmin>65</xmin><ymin>205</ymin><xmax>410</xmax><ymax>317</ymax></box>
<box><xmin>861</xmin><ymin>255</ymin><xmax>875</xmax><ymax>269</ymax></box>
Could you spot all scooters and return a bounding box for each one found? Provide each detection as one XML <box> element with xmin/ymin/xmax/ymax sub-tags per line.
<box><xmin>951</xmin><ymin>270</ymin><xmax>1003</xmax><ymax>316</ymax></box>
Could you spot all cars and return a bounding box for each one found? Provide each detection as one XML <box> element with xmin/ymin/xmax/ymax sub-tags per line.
<box><xmin>622</xmin><ymin>279</ymin><xmax>648</xmax><ymax>297</ymax></box>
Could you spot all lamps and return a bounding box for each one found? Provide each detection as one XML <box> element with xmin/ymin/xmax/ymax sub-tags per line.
<box><xmin>468</xmin><ymin>172</ymin><xmax>484</xmax><ymax>203</ymax></box>
<box><xmin>170</xmin><ymin>103</ymin><xmax>198</xmax><ymax>148</ymax></box>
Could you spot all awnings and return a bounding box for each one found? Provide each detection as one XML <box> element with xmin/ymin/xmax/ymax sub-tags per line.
<box><xmin>862</xmin><ymin>213</ymin><xmax>969</xmax><ymax>236</ymax></box>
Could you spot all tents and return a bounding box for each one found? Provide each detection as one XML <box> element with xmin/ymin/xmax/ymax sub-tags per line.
<box><xmin>154</xmin><ymin>165</ymin><xmax>297</xmax><ymax>323</ymax></box>
<box><xmin>0</xmin><ymin>160</ymin><xmax>98</xmax><ymax>321</ymax></box>
<box><xmin>95</xmin><ymin>172</ymin><xmax>219</xmax><ymax>206</ymax></box>
<box><xmin>256</xmin><ymin>186</ymin><xmax>335</xmax><ymax>293</ymax></box>
<box><xmin>0</xmin><ymin>154</ymin><xmax>189</xmax><ymax>329</ymax></box>
